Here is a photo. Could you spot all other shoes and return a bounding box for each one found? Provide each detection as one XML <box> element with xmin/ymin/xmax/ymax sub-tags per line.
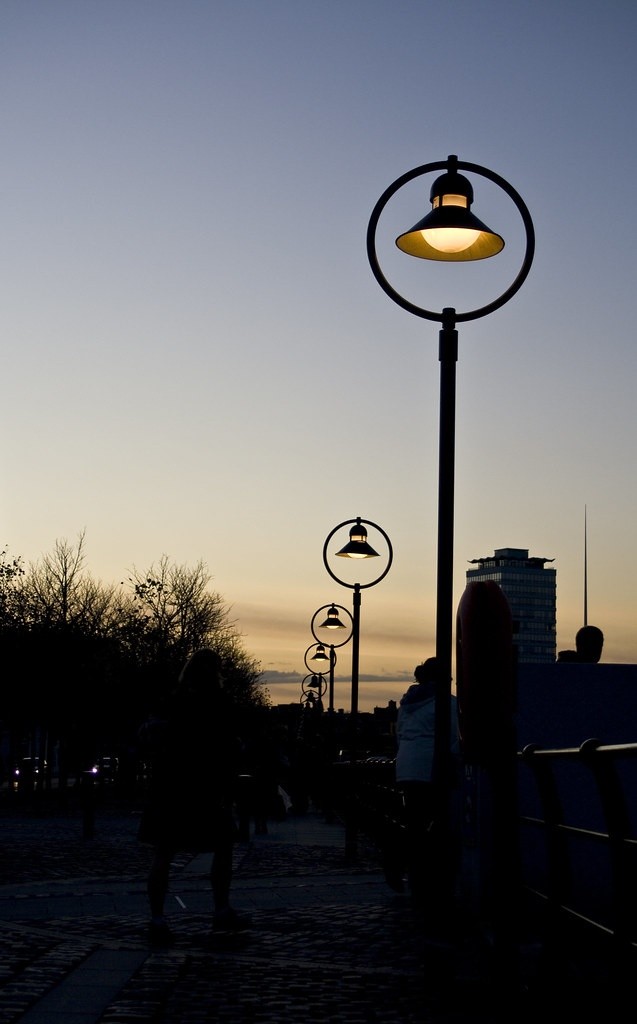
<box><xmin>150</xmin><ymin>919</ymin><xmax>170</xmax><ymax>933</ymax></box>
<box><xmin>215</xmin><ymin>907</ymin><xmax>248</xmax><ymax>929</ymax></box>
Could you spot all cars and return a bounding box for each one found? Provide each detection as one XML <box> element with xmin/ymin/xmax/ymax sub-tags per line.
<box><xmin>14</xmin><ymin>756</ymin><xmax>47</xmax><ymax>778</ymax></box>
<box><xmin>93</xmin><ymin>757</ymin><xmax>119</xmax><ymax>776</ymax></box>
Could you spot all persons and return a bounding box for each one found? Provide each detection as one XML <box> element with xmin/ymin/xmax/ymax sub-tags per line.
<box><xmin>395</xmin><ymin>659</ymin><xmax>458</xmax><ymax>910</ymax></box>
<box><xmin>142</xmin><ymin>648</ymin><xmax>250</xmax><ymax>935</ymax></box>
<box><xmin>557</xmin><ymin>626</ymin><xmax>603</xmax><ymax>663</ymax></box>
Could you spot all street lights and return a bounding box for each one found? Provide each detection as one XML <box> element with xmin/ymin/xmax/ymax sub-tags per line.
<box><xmin>304</xmin><ymin>643</ymin><xmax>337</xmax><ymax>713</ymax></box>
<box><xmin>321</xmin><ymin>517</ymin><xmax>393</xmax><ymax>769</ymax></box>
<box><xmin>363</xmin><ymin>153</ymin><xmax>536</xmax><ymax>832</ymax></box>
<box><xmin>310</xmin><ymin>602</ymin><xmax>354</xmax><ymax>724</ymax></box>
